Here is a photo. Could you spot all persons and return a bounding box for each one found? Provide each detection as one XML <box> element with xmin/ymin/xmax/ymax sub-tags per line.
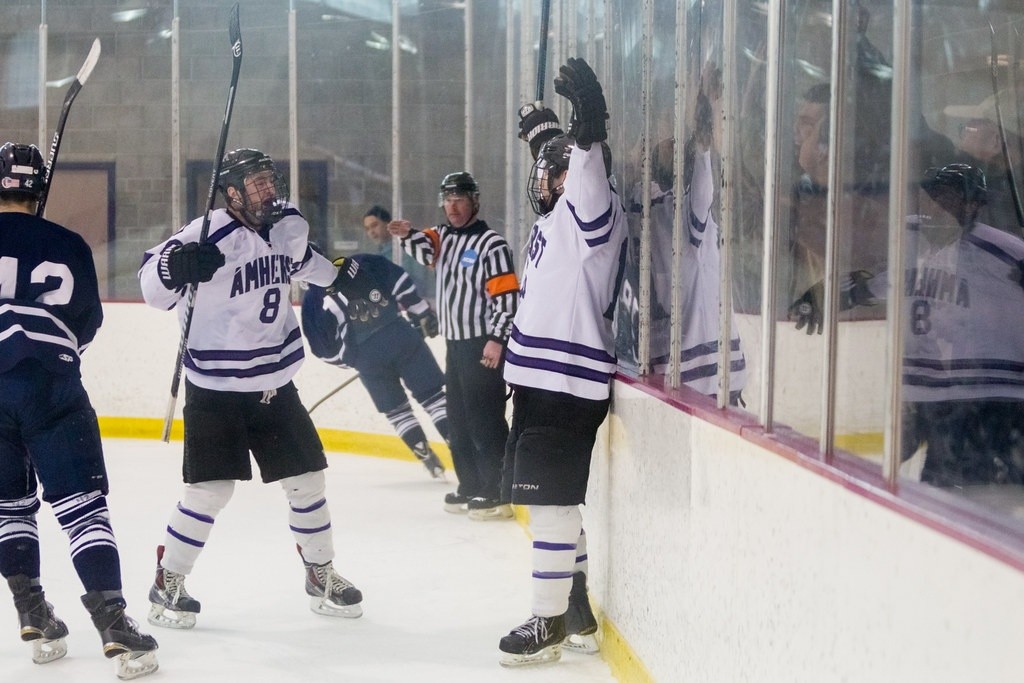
<box><xmin>138</xmin><ymin>148</ymin><xmax>388</xmax><ymax>631</ymax></box>
<box><xmin>363</xmin><ymin>205</ymin><xmax>437</xmax><ymax>300</ymax></box>
<box><xmin>387</xmin><ymin>171</ymin><xmax>520</xmax><ymax>523</ymax></box>
<box><xmin>498</xmin><ymin>57</ymin><xmax>628</xmax><ymax>666</ymax></box>
<box><xmin>295</xmin><ymin>241</ymin><xmax>452</xmax><ymax>485</ymax></box>
<box><xmin>628</xmin><ymin>63</ymin><xmax>748</xmax><ymax>410</ymax></box>
<box><xmin>788</xmin><ymin>0</ymin><xmax>1024</xmax><ymax>527</ymax></box>
<box><xmin>0</xmin><ymin>141</ymin><xmax>159</xmax><ymax>681</ymax></box>
<box><xmin>784</xmin><ymin>163</ymin><xmax>1024</xmax><ymax>485</ymax></box>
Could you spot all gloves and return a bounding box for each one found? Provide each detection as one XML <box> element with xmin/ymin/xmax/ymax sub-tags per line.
<box><xmin>518</xmin><ymin>103</ymin><xmax>555</xmax><ymax>142</ymax></box>
<box><xmin>554</xmin><ymin>57</ymin><xmax>609</xmax><ymax>145</ymax></box>
<box><xmin>167</xmin><ymin>241</ymin><xmax>225</xmax><ymax>286</ymax></box>
<box><xmin>407</xmin><ymin>299</ymin><xmax>439</xmax><ymax>339</ymax></box>
<box><xmin>331</xmin><ymin>257</ymin><xmax>389</xmax><ymax>321</ymax></box>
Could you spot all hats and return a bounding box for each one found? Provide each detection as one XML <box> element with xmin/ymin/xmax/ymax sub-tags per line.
<box><xmin>363</xmin><ymin>205</ymin><xmax>392</xmax><ymax>223</ymax></box>
<box><xmin>943</xmin><ymin>86</ymin><xmax>1024</xmax><ymax>138</ymax></box>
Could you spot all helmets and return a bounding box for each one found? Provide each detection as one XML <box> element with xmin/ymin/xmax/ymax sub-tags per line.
<box><xmin>0</xmin><ymin>141</ymin><xmax>47</xmax><ymax>202</ymax></box>
<box><xmin>527</xmin><ymin>133</ymin><xmax>612</xmax><ymax>217</ymax></box>
<box><xmin>215</xmin><ymin>147</ymin><xmax>288</xmax><ymax>224</ymax></box>
<box><xmin>440</xmin><ymin>172</ymin><xmax>481</xmax><ymax>197</ymax></box>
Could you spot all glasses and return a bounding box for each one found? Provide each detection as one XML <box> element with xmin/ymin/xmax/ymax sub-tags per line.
<box><xmin>958</xmin><ymin>122</ymin><xmax>996</xmax><ymax>140</ymax></box>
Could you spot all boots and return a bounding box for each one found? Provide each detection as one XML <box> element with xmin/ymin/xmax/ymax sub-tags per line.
<box><xmin>81</xmin><ymin>589</ymin><xmax>159</xmax><ymax>659</ymax></box>
<box><xmin>148</xmin><ymin>545</ymin><xmax>201</xmax><ymax>612</ymax></box>
<box><xmin>7</xmin><ymin>573</ymin><xmax>69</xmax><ymax>639</ymax></box>
<box><xmin>297</xmin><ymin>542</ymin><xmax>363</xmax><ymax>605</ymax></box>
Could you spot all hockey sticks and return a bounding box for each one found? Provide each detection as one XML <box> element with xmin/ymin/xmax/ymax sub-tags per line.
<box><xmin>534</xmin><ymin>0</ymin><xmax>551</xmax><ymax>114</ymax></box>
<box><xmin>37</xmin><ymin>35</ymin><xmax>101</xmax><ymax>219</ymax></box>
<box><xmin>308</xmin><ymin>372</ymin><xmax>360</xmax><ymax>416</ymax></box>
<box><xmin>160</xmin><ymin>3</ymin><xmax>244</xmax><ymax>445</ymax></box>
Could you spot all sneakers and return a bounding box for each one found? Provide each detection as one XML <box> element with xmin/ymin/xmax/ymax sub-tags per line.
<box><xmin>467</xmin><ymin>484</ymin><xmax>516</xmax><ymax>509</ymax></box>
<box><xmin>444</xmin><ymin>479</ymin><xmax>474</xmax><ymax>504</ymax></box>
<box><xmin>561</xmin><ymin>571</ymin><xmax>599</xmax><ymax>635</ymax></box>
<box><xmin>413</xmin><ymin>439</ymin><xmax>444</xmax><ymax>480</ymax></box>
<box><xmin>498</xmin><ymin>612</ymin><xmax>566</xmax><ymax>654</ymax></box>
